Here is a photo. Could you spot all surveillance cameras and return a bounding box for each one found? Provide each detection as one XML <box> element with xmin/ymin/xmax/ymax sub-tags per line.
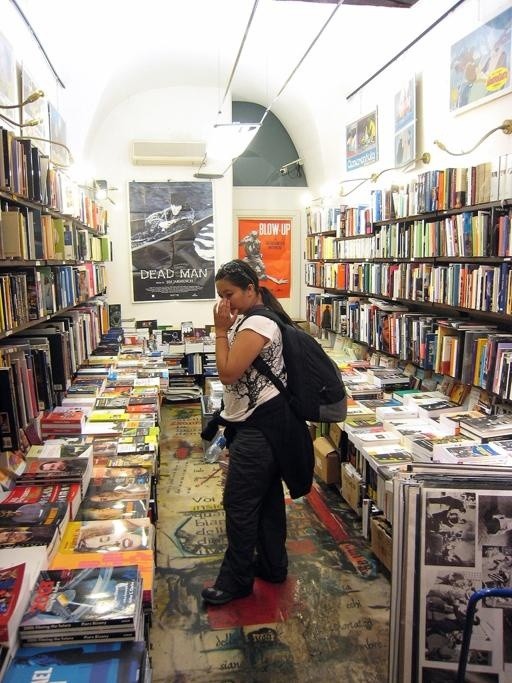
<box><xmin>280</xmin><ymin>161</ymin><xmax>298</xmax><ymax>174</ymax></box>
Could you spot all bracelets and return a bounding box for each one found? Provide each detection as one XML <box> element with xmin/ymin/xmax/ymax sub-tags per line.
<box><xmin>213</xmin><ymin>333</ymin><xmax>228</xmax><ymax>339</ymax></box>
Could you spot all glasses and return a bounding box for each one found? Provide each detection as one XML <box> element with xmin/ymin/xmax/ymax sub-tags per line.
<box><xmin>218</xmin><ymin>263</ymin><xmax>253</xmax><ymax>284</ymax></box>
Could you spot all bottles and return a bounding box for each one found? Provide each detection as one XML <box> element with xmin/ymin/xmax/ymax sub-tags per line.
<box><xmin>204</xmin><ymin>429</ymin><xmax>227</xmax><ymax>465</ymax></box>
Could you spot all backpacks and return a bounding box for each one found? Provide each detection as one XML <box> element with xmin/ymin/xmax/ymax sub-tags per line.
<box><xmin>235</xmin><ymin>308</ymin><xmax>346</xmax><ymax>423</ymax></box>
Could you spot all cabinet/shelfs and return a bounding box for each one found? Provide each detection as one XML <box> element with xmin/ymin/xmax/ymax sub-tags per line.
<box><xmin>305</xmin><ymin>157</ymin><xmax>512</xmax><ymax>683</ymax></box>
<box><xmin>0</xmin><ymin>46</ymin><xmax>271</xmax><ymax>680</ymax></box>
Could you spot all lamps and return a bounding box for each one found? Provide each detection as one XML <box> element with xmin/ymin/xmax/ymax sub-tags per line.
<box><xmin>186</xmin><ymin>124</ymin><xmax>258</xmax><ymax>175</ymax></box>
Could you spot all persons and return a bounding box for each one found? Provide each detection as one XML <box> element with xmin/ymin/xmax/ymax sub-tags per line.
<box><xmin>419</xmin><ymin>492</ymin><xmax>511</xmax><ymax>666</ymax></box>
<box><xmin>202</xmin><ymin>255</ymin><xmax>293</xmax><ymax>599</ymax></box>
<box><xmin>256</xmin><ymin>273</ymin><xmax>288</xmax><ymax>284</ymax></box>
<box><xmin>239</xmin><ymin>230</ymin><xmax>267</xmax><ymax>274</ymax></box>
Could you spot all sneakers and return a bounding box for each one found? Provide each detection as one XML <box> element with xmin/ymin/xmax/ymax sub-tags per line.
<box><xmin>253</xmin><ymin>553</ymin><xmax>286</xmax><ymax>583</ymax></box>
<box><xmin>201</xmin><ymin>585</ymin><xmax>253</xmax><ymax>605</ymax></box>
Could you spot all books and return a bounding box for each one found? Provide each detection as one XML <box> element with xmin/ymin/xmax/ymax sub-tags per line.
<box><xmin>1</xmin><ymin>128</ymin><xmax>114</xmax><ymax>332</ymax></box>
<box><xmin>299</xmin><ymin>158</ymin><xmax>511</xmax><ymax>543</ymax></box>
<box><xmin>0</xmin><ymin>318</ymin><xmax>223</xmax><ymax>683</ymax></box>
